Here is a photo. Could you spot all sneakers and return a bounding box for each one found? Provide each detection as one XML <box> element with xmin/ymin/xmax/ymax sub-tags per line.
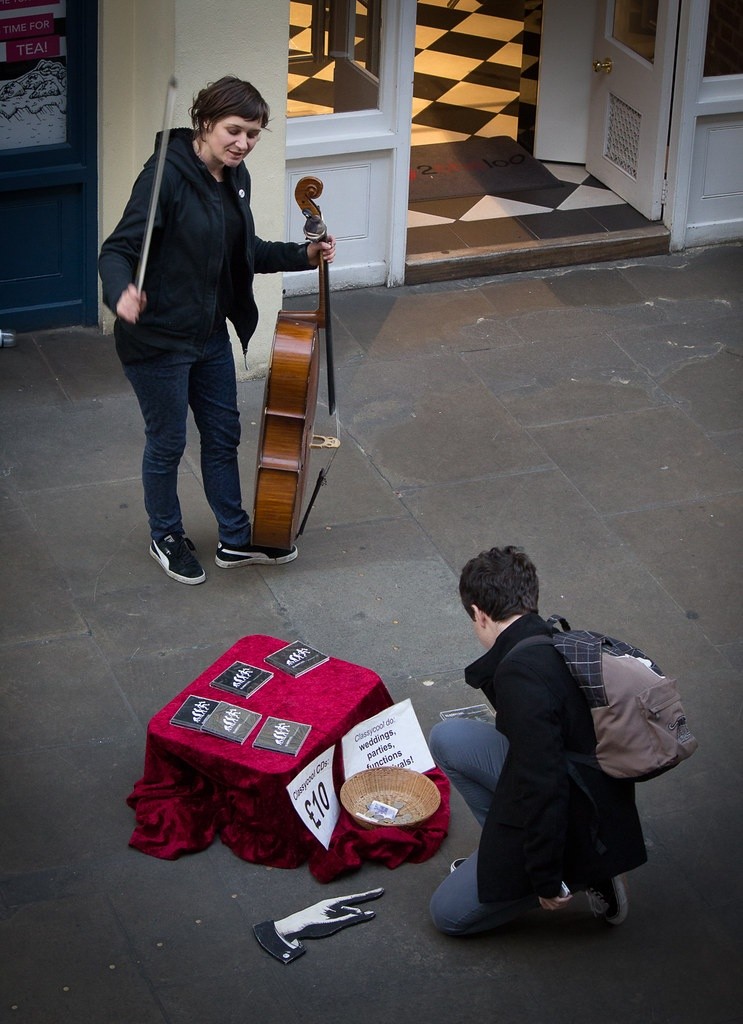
<box><xmin>450</xmin><ymin>855</ymin><xmax>468</xmax><ymax>874</ymax></box>
<box><xmin>214</xmin><ymin>537</ymin><xmax>300</xmax><ymax>570</ymax></box>
<box><xmin>148</xmin><ymin>530</ymin><xmax>208</xmax><ymax>585</ymax></box>
<box><xmin>584</xmin><ymin>875</ymin><xmax>630</xmax><ymax>926</ymax></box>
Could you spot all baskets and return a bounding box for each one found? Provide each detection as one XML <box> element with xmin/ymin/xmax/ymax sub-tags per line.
<box><xmin>337</xmin><ymin>766</ymin><xmax>443</xmax><ymax>828</ymax></box>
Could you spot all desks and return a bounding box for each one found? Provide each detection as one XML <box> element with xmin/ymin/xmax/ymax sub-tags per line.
<box><xmin>144</xmin><ymin>634</ymin><xmax>392</xmax><ymax>868</ymax></box>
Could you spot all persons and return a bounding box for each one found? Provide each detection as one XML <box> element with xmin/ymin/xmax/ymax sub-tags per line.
<box><xmin>99</xmin><ymin>76</ymin><xmax>337</xmax><ymax>586</ymax></box>
<box><xmin>426</xmin><ymin>543</ymin><xmax>648</xmax><ymax>940</ymax></box>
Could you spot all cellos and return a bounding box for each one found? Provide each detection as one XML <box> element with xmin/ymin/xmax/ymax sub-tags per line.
<box><xmin>254</xmin><ymin>174</ymin><xmax>345</xmax><ymax>554</ymax></box>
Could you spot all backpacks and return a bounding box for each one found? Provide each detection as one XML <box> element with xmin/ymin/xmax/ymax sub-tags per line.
<box><xmin>545</xmin><ymin>614</ymin><xmax>700</xmax><ymax>784</ymax></box>
